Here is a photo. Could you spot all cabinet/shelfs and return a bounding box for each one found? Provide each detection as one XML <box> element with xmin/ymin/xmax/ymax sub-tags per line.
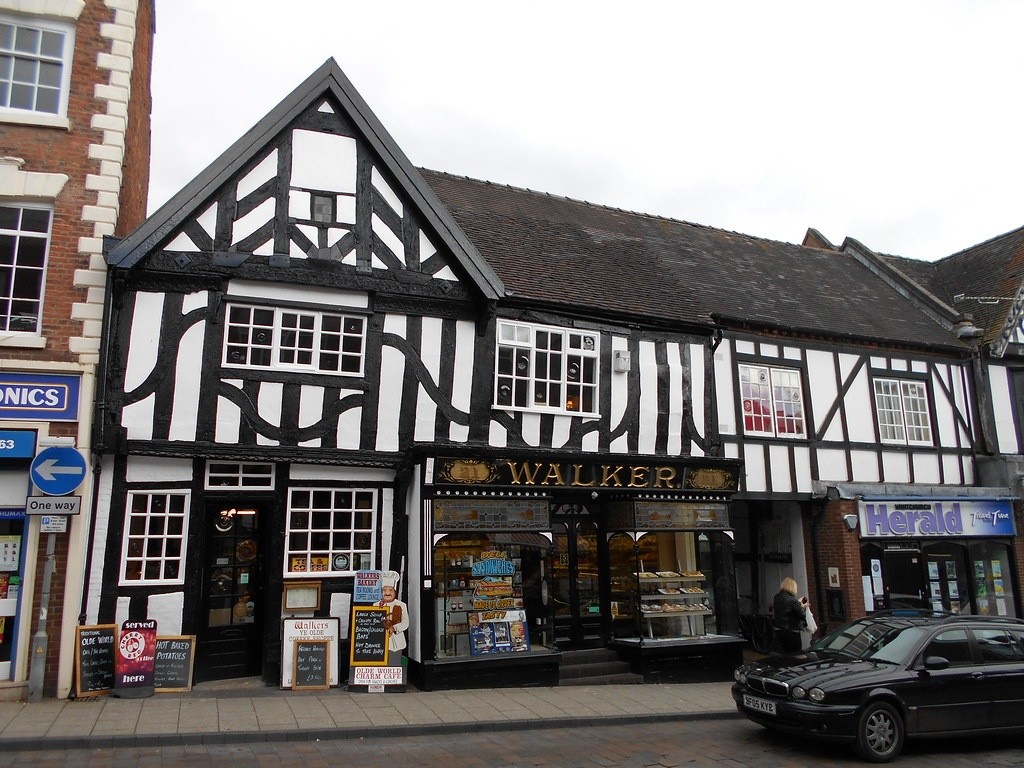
<box><xmin>432</xmin><ymin>539</ymin><xmax>527</xmax><ymax>653</ymax></box>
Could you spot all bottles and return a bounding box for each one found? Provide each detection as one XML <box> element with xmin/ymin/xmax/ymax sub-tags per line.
<box><xmin>459</xmin><ymin>577</ymin><xmax>465</xmax><ymax>587</ymax></box>
<box><xmin>451</xmin><ymin>575</ymin><xmax>456</xmax><ymax>589</ymax></box>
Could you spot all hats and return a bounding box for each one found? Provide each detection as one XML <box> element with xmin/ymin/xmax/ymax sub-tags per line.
<box><xmin>378</xmin><ymin>570</ymin><xmax>401</xmax><ymax>591</ymax></box>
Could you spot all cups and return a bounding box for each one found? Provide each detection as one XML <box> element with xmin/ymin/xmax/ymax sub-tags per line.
<box><xmin>458</xmin><ymin>601</ymin><xmax>464</xmax><ymax>610</ymax></box>
<box><xmin>451</xmin><ymin>601</ymin><xmax>456</xmax><ymax>610</ymax></box>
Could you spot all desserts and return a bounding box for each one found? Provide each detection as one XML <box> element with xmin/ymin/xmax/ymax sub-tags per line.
<box><xmin>640</xmin><ymin>570</ymin><xmax>705</xmax><ymax>610</ymax></box>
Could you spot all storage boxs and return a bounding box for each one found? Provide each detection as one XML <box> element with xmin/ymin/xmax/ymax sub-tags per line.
<box><xmin>0</xmin><ymin>574</ymin><xmax>9</xmax><ymax>586</ymax></box>
<box><xmin>7</xmin><ymin>586</ymin><xmax>18</xmax><ymax>599</ymax></box>
<box><xmin>359</xmin><ymin>554</ymin><xmax>372</xmax><ymax>571</ymax></box>
<box><xmin>311</xmin><ymin>558</ymin><xmax>329</xmax><ymax>572</ymax></box>
<box><xmin>289</xmin><ymin>558</ymin><xmax>307</xmax><ymax>572</ymax></box>
<box><xmin>0</xmin><ymin>535</ymin><xmax>21</xmax><ymax>572</ymax></box>
<box><xmin>0</xmin><ymin>587</ymin><xmax>8</xmax><ymax>598</ymax></box>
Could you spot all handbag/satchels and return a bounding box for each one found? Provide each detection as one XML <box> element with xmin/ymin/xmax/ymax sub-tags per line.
<box><xmin>806</xmin><ymin>606</ymin><xmax>818</xmax><ymax>634</ymax></box>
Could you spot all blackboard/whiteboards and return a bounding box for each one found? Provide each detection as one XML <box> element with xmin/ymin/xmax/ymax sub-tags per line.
<box><xmin>281</xmin><ymin>616</ymin><xmax>340</xmax><ymax>689</ymax></box>
<box><xmin>153</xmin><ymin>634</ymin><xmax>196</xmax><ymax>692</ymax></box>
<box><xmin>292</xmin><ymin>639</ymin><xmax>331</xmax><ymax>690</ymax></box>
<box><xmin>76</xmin><ymin>623</ymin><xmax>119</xmax><ymax>696</ymax></box>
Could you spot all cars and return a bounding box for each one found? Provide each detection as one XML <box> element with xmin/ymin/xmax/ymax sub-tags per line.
<box><xmin>732</xmin><ymin>608</ymin><xmax>1024</xmax><ymax>763</ymax></box>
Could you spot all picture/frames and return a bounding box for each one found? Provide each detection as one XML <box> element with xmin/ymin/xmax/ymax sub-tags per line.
<box><xmin>75</xmin><ymin>623</ymin><xmax>120</xmax><ymax>697</ymax></box>
<box><xmin>154</xmin><ymin>634</ymin><xmax>197</xmax><ymax>692</ymax></box>
<box><xmin>294</xmin><ymin>640</ymin><xmax>330</xmax><ymax>690</ymax></box>
